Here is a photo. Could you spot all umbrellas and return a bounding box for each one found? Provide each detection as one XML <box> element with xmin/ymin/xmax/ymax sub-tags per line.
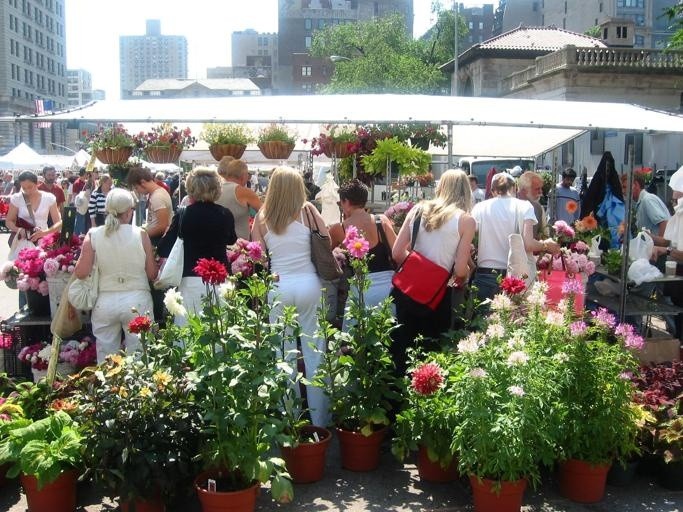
<box><xmin>0</xmin><ymin>142</ymin><xmax>55</xmax><ymax>172</ymax></box>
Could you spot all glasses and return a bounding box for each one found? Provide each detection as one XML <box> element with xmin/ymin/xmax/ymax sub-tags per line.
<box><xmin>337</xmin><ymin>201</ymin><xmax>341</xmax><ymax>206</ymax></box>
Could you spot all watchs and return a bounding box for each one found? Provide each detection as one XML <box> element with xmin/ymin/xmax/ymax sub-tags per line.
<box><xmin>666</xmin><ymin>246</ymin><xmax>672</xmax><ymax>257</ymax></box>
<box><xmin>541</xmin><ymin>241</ymin><xmax>548</xmax><ymax>254</ymax></box>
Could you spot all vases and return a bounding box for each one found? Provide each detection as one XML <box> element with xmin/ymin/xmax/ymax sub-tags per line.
<box><xmin>147</xmin><ymin>144</ymin><xmax>183</xmax><ymax>170</ymax></box>
<box><xmin>273</xmin><ymin>419</ymin><xmax>335</xmax><ymax>485</ymax></box>
<box><xmin>409</xmin><ymin>439</ymin><xmax>458</xmax><ymax>488</ymax></box>
<box><xmin>611</xmin><ymin>454</ymin><xmax>639</xmax><ymax>485</ymax></box>
<box><xmin>328</xmin><ymin>414</ymin><xmax>386</xmax><ymax>475</ymax></box>
<box><xmin>194</xmin><ymin>466</ymin><xmax>261</xmax><ymax>512</ymax></box>
<box><xmin>21</xmin><ymin>287</ymin><xmax>52</xmax><ymax>322</ymax></box>
<box><xmin>467</xmin><ymin>469</ymin><xmax>532</xmax><ymax>512</ymax></box>
<box><xmin>410</xmin><ymin>134</ymin><xmax>430</xmax><ymax>151</ymax></box>
<box><xmin>649</xmin><ymin>451</ymin><xmax>683</xmax><ymax>491</ymax></box>
<box><xmin>559</xmin><ymin>448</ymin><xmax>617</xmax><ymax>505</ymax></box>
<box><xmin>16</xmin><ymin>465</ymin><xmax>79</xmax><ymax>512</ymax></box>
<box><xmin>325</xmin><ymin>139</ymin><xmax>355</xmax><ymax>161</ymax></box>
<box><xmin>116</xmin><ymin>484</ymin><xmax>176</xmax><ymax>512</ymax></box>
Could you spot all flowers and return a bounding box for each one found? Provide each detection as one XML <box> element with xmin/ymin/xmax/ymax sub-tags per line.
<box><xmin>381</xmin><ymin>201</ymin><xmax>412</xmax><ymax>229</ymax></box>
<box><xmin>531</xmin><ymin>202</ymin><xmax>599</xmax><ymax>275</ymax></box>
<box><xmin>131</xmin><ymin>123</ymin><xmax>197</xmax><ymax>152</ymax></box>
<box><xmin>0</xmin><ymin>236</ymin><xmax>683</xmax><ymax>476</ymax></box>
<box><xmin>307</xmin><ymin>122</ymin><xmax>451</xmax><ymax>185</ymax></box>
<box><xmin>79</xmin><ymin>119</ymin><xmax>137</xmax><ymax>149</ymax></box>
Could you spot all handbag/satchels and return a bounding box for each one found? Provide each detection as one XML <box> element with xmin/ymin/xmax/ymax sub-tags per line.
<box><xmin>303</xmin><ymin>203</ymin><xmax>342</xmax><ymax>282</ymax></box>
<box><xmin>390</xmin><ymin>201</ymin><xmax>455</xmax><ymax>311</ymax></box>
<box><xmin>506</xmin><ymin>200</ymin><xmax>539</xmax><ymax>304</ymax></box>
<box><xmin>539</xmin><ymin>251</ymin><xmax>585</xmax><ymax>319</ymax></box>
<box><xmin>67</xmin><ymin>233</ymin><xmax>102</xmax><ymax>314</ymax></box>
<box><xmin>151</xmin><ymin>205</ymin><xmax>185</xmax><ymax>292</ymax></box>
<box><xmin>6</xmin><ymin>193</ymin><xmax>35</xmax><ymax>251</ymax></box>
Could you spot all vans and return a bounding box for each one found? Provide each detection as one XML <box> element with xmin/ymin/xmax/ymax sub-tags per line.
<box><xmin>459</xmin><ymin>157</ymin><xmax>536</xmax><ymax>195</ymax></box>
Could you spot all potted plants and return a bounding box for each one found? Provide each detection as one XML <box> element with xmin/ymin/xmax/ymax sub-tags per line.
<box><xmin>533</xmin><ymin>168</ymin><xmax>551</xmax><ymax>206</ymax></box>
<box><xmin>253</xmin><ymin>121</ymin><xmax>301</xmax><ymax>161</ymax></box>
<box><xmin>604</xmin><ymin>247</ymin><xmax>632</xmax><ymax>280</ymax></box>
<box><xmin>200</xmin><ymin>118</ymin><xmax>258</xmax><ymax>161</ymax></box>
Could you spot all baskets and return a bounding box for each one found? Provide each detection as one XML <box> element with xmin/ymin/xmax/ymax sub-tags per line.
<box><xmin>256</xmin><ymin>142</ymin><xmax>296</xmax><ymax>159</ymax></box>
<box><xmin>209</xmin><ymin>144</ymin><xmax>247</xmax><ymax>162</ymax></box>
<box><xmin>410</xmin><ymin>136</ymin><xmax>429</xmax><ymax>150</ymax></box>
<box><xmin>139</xmin><ymin>147</ymin><xmax>183</xmax><ymax>163</ymax></box>
<box><xmin>318</xmin><ymin>142</ymin><xmax>355</xmax><ymax>158</ymax></box>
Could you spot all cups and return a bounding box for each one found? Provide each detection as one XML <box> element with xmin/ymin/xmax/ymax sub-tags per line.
<box><xmin>665</xmin><ymin>260</ymin><xmax>677</xmax><ymax>279</ymax></box>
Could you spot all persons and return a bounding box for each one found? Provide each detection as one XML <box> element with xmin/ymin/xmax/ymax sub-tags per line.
<box><xmin>128</xmin><ymin>165</ymin><xmax>174</xmax><ymax>244</ymax></box>
<box><xmin>74</xmin><ymin>188</ymin><xmax>158</xmax><ymax>368</ymax></box>
<box><xmin>329</xmin><ymin>180</ymin><xmax>398</xmax><ymax>355</ymax></box>
<box><xmin>621</xmin><ymin>170</ymin><xmax>673</xmax><ymax>274</ymax></box>
<box><xmin>38</xmin><ymin>167</ymin><xmax>65</xmax><ymax>227</ymax></box>
<box><xmin>548</xmin><ymin>168</ymin><xmax>580</xmax><ymax>230</ymax></box>
<box><xmin>214</xmin><ymin>158</ymin><xmax>263</xmax><ymax>239</ymax></box>
<box><xmin>91</xmin><ymin>166</ymin><xmax>101</xmax><ymax>182</ymax></box>
<box><xmin>468</xmin><ymin>175</ymin><xmax>486</xmax><ymax>203</ymax></box>
<box><xmin>463</xmin><ymin>172</ymin><xmax>562</xmax><ymax>336</ymax></box>
<box><xmin>250</xmin><ymin>167</ymin><xmax>333</xmax><ymax>430</ymax></box>
<box><xmin>517</xmin><ymin>171</ymin><xmax>544</xmax><ymax>255</ymax></box>
<box><xmin>0</xmin><ymin>174</ymin><xmax>12</xmax><ymax>196</ymax></box>
<box><xmin>62</xmin><ymin>175</ymin><xmax>77</xmax><ymax>237</ymax></box>
<box><xmin>4</xmin><ymin>172</ymin><xmax>63</xmax><ymax>310</ymax></box>
<box><xmin>170</xmin><ymin>173</ymin><xmax>180</xmax><ymax>210</ymax></box>
<box><xmin>391</xmin><ymin>169</ymin><xmax>476</xmax><ymax>335</ymax></box>
<box><xmin>172</xmin><ymin>174</ymin><xmax>188</xmax><ymax>200</ymax></box>
<box><xmin>640</xmin><ymin>165</ymin><xmax>683</xmax><ymax>340</ymax></box>
<box><xmin>157</xmin><ymin>169</ymin><xmax>237</xmax><ymax>356</ymax></box>
<box><xmin>72</xmin><ymin>168</ymin><xmax>88</xmax><ymax>236</ymax></box>
<box><xmin>218</xmin><ymin>155</ymin><xmax>236</xmax><ymax>182</ymax></box>
<box><xmin>88</xmin><ymin>175</ymin><xmax>112</xmax><ymax>227</ymax></box>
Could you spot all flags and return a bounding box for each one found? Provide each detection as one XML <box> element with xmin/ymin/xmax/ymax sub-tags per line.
<box><xmin>33</xmin><ymin>99</ymin><xmax>53</xmax><ymax>129</ymax></box>
